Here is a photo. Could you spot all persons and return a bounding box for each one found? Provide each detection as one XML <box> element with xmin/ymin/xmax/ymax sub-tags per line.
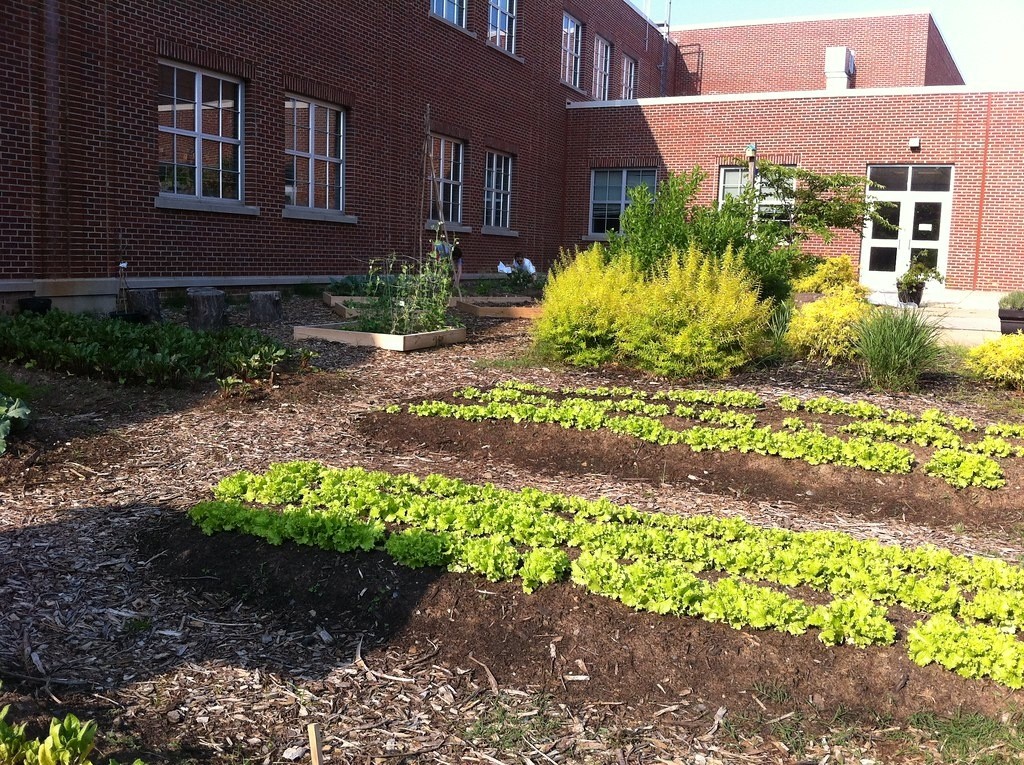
<box><xmin>432</xmin><ymin>241</ymin><xmax>462</xmax><ymax>282</ymax></box>
<box><xmin>507</xmin><ymin>252</ymin><xmax>536</xmax><ymax>282</ymax></box>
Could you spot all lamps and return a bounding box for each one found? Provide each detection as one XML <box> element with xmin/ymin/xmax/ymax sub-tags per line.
<box><xmin>909</xmin><ymin>138</ymin><xmax>921</xmax><ymax>147</ymax></box>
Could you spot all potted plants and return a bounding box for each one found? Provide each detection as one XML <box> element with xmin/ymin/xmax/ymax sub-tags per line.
<box><xmin>999</xmin><ymin>290</ymin><xmax>1024</xmax><ymax>335</ymax></box>
<box><xmin>897</xmin><ymin>249</ymin><xmax>950</xmax><ymax>308</ymax></box>
